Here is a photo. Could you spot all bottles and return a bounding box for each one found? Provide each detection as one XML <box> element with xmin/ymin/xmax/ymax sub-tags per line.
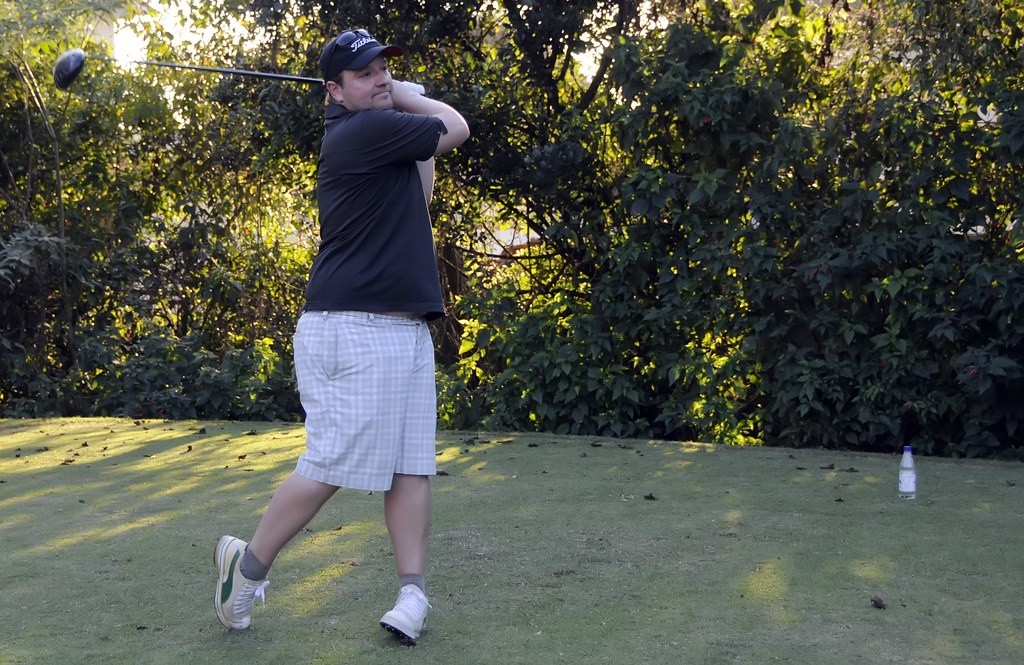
<box><xmin>898</xmin><ymin>446</ymin><xmax>918</xmax><ymax>500</ymax></box>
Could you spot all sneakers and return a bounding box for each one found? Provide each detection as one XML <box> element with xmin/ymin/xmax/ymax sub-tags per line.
<box><xmin>212</xmin><ymin>535</ymin><xmax>271</xmax><ymax>629</ymax></box>
<box><xmin>379</xmin><ymin>585</ymin><xmax>432</xmax><ymax>639</ymax></box>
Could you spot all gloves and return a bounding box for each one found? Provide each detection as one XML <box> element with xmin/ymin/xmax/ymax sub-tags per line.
<box><xmin>401</xmin><ymin>79</ymin><xmax>426</xmax><ymax>96</ymax></box>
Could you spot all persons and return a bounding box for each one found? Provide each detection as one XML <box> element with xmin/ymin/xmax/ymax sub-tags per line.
<box><xmin>214</xmin><ymin>30</ymin><xmax>471</xmax><ymax>642</ymax></box>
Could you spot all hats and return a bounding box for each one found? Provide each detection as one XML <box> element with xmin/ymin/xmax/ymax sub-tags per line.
<box><xmin>318</xmin><ymin>32</ymin><xmax>400</xmax><ymax>80</ymax></box>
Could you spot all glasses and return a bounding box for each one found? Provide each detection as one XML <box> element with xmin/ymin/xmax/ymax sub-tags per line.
<box><xmin>322</xmin><ymin>28</ymin><xmax>371</xmax><ymax>70</ymax></box>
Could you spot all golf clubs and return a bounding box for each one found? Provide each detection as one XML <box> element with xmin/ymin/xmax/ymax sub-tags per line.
<box><xmin>52</xmin><ymin>47</ymin><xmax>423</xmax><ymax>129</ymax></box>
